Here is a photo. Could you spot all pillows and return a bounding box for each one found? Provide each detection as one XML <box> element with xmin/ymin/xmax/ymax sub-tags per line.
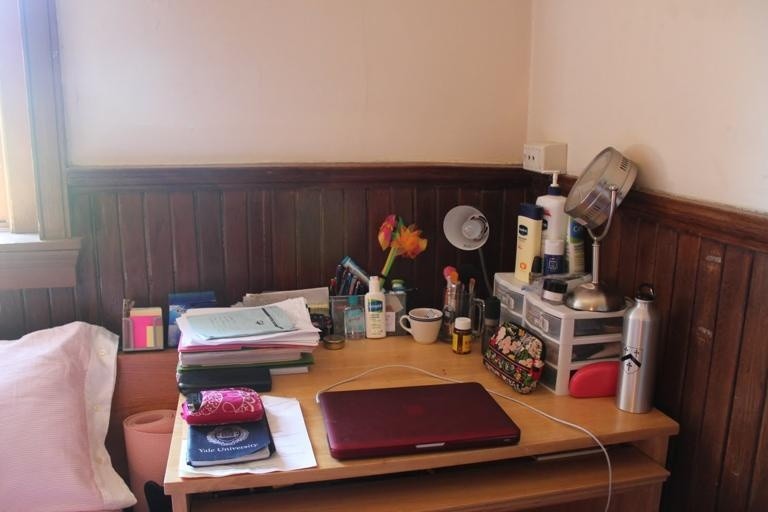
<box><xmin>0</xmin><ymin>320</ymin><xmax>137</xmax><ymax>512</ymax></box>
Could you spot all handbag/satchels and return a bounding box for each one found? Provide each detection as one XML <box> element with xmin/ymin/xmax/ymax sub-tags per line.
<box><xmin>483</xmin><ymin>321</ymin><xmax>544</xmax><ymax>395</ymax></box>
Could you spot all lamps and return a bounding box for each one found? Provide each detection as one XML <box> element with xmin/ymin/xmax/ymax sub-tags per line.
<box><xmin>443</xmin><ymin>205</ymin><xmax>501</xmax><ymax>326</ymax></box>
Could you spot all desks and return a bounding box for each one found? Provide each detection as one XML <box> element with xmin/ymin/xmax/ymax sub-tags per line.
<box><xmin>164</xmin><ymin>331</ymin><xmax>684</xmax><ymax>510</ymax></box>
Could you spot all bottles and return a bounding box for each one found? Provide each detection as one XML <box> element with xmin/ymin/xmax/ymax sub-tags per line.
<box><xmin>452</xmin><ymin>316</ymin><xmax>472</xmax><ymax>354</ymax></box>
<box><xmin>615</xmin><ymin>283</ymin><xmax>660</xmax><ymax>414</ymax></box>
<box><xmin>482</xmin><ymin>318</ymin><xmax>500</xmax><ymax>355</ymax></box>
<box><xmin>343</xmin><ymin>276</ymin><xmax>408</xmax><ymax>340</ymax></box>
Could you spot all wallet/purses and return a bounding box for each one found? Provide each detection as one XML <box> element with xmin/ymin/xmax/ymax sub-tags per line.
<box><xmin>181</xmin><ymin>387</ymin><xmax>264</xmax><ymax>426</ymax></box>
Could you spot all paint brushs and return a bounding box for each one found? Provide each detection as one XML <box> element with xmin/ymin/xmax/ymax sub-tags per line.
<box><xmin>442</xmin><ymin>266</ymin><xmax>475</xmax><ymax>335</ymax></box>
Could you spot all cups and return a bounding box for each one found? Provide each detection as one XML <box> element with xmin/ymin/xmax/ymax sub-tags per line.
<box><xmin>444</xmin><ymin>287</ymin><xmax>486</xmax><ymax>342</ymax></box>
<box><xmin>399</xmin><ymin>307</ymin><xmax>444</xmax><ymax>345</ymax></box>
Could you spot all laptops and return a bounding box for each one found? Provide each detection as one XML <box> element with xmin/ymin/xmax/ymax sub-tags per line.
<box><xmin>319</xmin><ymin>381</ymin><xmax>521</xmax><ymax>460</ymax></box>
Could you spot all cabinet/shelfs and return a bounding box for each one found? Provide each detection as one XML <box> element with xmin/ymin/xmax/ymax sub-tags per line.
<box><xmin>493</xmin><ymin>272</ymin><xmax>632</xmax><ymax>396</ymax></box>
<box><xmin>109</xmin><ymin>349</ymin><xmax>180</xmax><ymax>462</ymax></box>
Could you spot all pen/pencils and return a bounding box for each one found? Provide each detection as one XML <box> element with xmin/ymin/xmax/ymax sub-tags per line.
<box><xmin>328</xmin><ymin>264</ymin><xmax>368</xmax><ymax>295</ymax></box>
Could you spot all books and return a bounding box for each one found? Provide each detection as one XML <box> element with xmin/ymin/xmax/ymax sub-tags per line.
<box><xmin>186</xmin><ymin>411</ymin><xmax>272</xmax><ymax>467</ymax></box>
<box><xmin>175</xmin><ymin>297</ymin><xmax>322</xmax><ymax>375</ymax></box>
